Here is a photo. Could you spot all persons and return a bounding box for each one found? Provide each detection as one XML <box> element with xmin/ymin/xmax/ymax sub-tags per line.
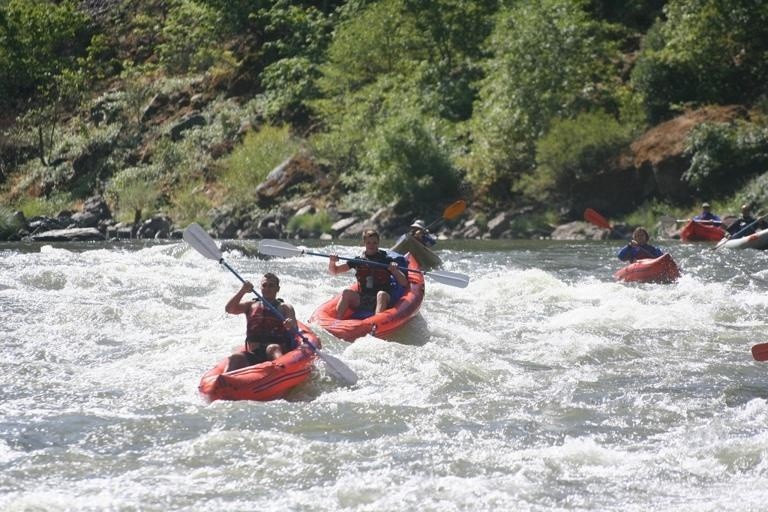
<box><xmin>224</xmin><ymin>272</ymin><xmax>298</xmax><ymax>374</ymax></box>
<box><xmin>693</xmin><ymin>202</ymin><xmax>722</xmax><ymax>227</ymax></box>
<box><xmin>407</xmin><ymin>220</ymin><xmax>436</xmax><ymax>248</ymax></box>
<box><xmin>618</xmin><ymin>227</ymin><xmax>664</xmax><ymax>262</ymax></box>
<box><xmin>330</xmin><ymin>231</ymin><xmax>410</xmax><ymax>319</ymax></box>
<box><xmin>725</xmin><ymin>204</ymin><xmax>768</xmax><ymax>240</ymax></box>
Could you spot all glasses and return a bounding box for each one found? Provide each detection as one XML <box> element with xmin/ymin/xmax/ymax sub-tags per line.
<box><xmin>261</xmin><ymin>283</ymin><xmax>277</xmax><ymax>288</ymax></box>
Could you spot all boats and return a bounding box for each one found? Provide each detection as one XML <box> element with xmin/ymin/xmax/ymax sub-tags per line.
<box><xmin>389</xmin><ymin>231</ymin><xmax>442</xmax><ymax>269</ymax></box>
<box><xmin>679</xmin><ymin>219</ymin><xmax>724</xmax><ymax>242</ymax></box>
<box><xmin>718</xmin><ymin>228</ymin><xmax>768</xmax><ymax>249</ymax></box>
<box><xmin>200</xmin><ymin>320</ymin><xmax>322</xmax><ymax>401</ymax></box>
<box><xmin>614</xmin><ymin>255</ymin><xmax>678</xmax><ymax>286</ymax></box>
<box><xmin>311</xmin><ymin>253</ymin><xmax>424</xmax><ymax>345</ymax></box>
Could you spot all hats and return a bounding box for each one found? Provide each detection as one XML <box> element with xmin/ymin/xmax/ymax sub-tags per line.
<box><xmin>409</xmin><ymin>219</ymin><xmax>426</xmax><ymax>231</ymax></box>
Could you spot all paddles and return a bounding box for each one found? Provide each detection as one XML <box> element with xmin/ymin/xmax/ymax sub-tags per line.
<box><xmin>259</xmin><ymin>238</ymin><xmax>469</xmax><ymax>288</ymax></box>
<box><xmin>663</xmin><ymin>216</ymin><xmax>736</xmax><ymax>227</ymax></box>
<box><xmin>711</xmin><ymin>213</ymin><xmax>768</xmax><ymax>250</ymax></box>
<box><xmin>183</xmin><ymin>222</ymin><xmax>358</xmax><ymax>386</ymax></box>
<box><xmin>584</xmin><ymin>208</ymin><xmax>656</xmax><ymax>258</ymax></box>
<box><xmin>426</xmin><ymin>200</ymin><xmax>465</xmax><ymax>229</ymax></box>
<box><xmin>752</xmin><ymin>343</ymin><xmax>768</xmax><ymax>361</ymax></box>
